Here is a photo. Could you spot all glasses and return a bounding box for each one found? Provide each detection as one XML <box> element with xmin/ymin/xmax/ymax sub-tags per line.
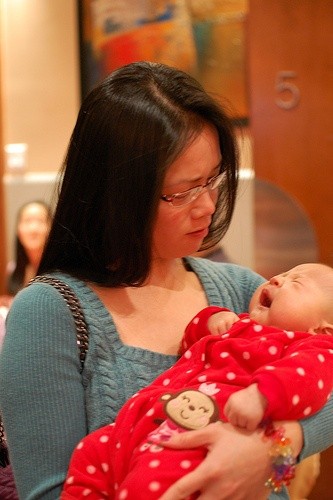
<box><xmin>151</xmin><ymin>167</ymin><xmax>230</xmax><ymax>208</ymax></box>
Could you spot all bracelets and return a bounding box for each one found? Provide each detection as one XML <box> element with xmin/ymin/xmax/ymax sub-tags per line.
<box><xmin>263</xmin><ymin>419</ymin><xmax>296</xmax><ymax>494</ymax></box>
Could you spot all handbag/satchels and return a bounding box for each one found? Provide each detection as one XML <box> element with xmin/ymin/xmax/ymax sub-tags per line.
<box><xmin>0</xmin><ymin>276</ymin><xmax>89</xmax><ymax>500</ymax></box>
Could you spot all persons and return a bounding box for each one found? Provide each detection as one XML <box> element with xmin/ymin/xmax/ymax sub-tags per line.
<box><xmin>0</xmin><ymin>200</ymin><xmax>53</xmax><ymax>308</ymax></box>
<box><xmin>0</xmin><ymin>62</ymin><xmax>333</xmax><ymax>500</ymax></box>
<box><xmin>55</xmin><ymin>263</ymin><xmax>333</xmax><ymax>500</ymax></box>
